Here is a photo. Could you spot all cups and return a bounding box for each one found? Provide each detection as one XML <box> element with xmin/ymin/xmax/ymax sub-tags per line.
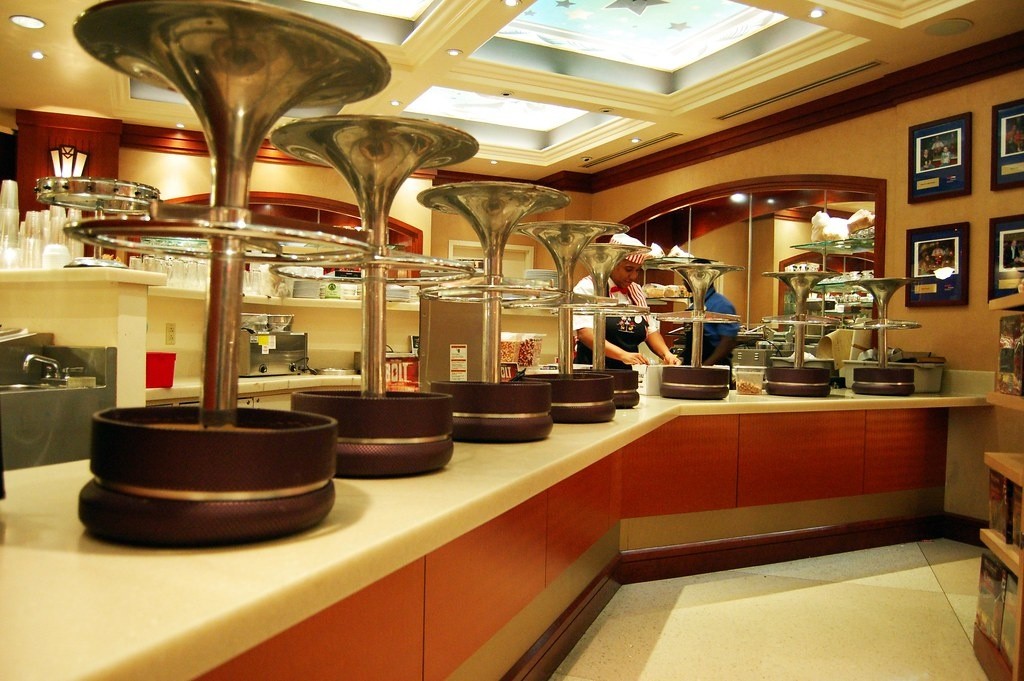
<box><xmin>130</xmin><ymin>255</ymin><xmax>262</xmax><ymax>297</ymax></box>
<box><xmin>43</xmin><ymin>244</ymin><xmax>70</xmax><ymax>269</ymax></box>
<box><xmin>341</xmin><ymin>284</ymin><xmax>357</xmax><ymax>298</ymax></box>
<box><xmin>0</xmin><ymin>180</ymin><xmax>85</xmax><ymax>269</ymax></box>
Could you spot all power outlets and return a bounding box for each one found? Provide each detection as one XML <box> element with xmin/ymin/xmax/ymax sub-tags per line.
<box><xmin>166</xmin><ymin>323</ymin><xmax>176</xmax><ymax>345</ymax></box>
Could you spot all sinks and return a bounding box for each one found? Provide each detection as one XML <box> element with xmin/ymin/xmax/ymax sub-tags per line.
<box><xmin>0</xmin><ymin>384</ymin><xmax>62</xmax><ymax>395</ymax></box>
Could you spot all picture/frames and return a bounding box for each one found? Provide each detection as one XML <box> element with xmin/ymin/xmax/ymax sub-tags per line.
<box><xmin>990</xmin><ymin>98</ymin><xmax>1024</xmax><ymax>191</ymax></box>
<box><xmin>904</xmin><ymin>222</ymin><xmax>970</xmax><ymax>308</ymax></box>
<box><xmin>908</xmin><ymin>112</ymin><xmax>973</xmax><ymax>204</ymax></box>
<box><xmin>987</xmin><ymin>214</ymin><xmax>1024</xmax><ymax>311</ymax></box>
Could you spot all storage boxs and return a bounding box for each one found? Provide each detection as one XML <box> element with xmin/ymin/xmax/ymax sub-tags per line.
<box><xmin>146</xmin><ymin>352</ymin><xmax>176</xmax><ymax>388</ymax></box>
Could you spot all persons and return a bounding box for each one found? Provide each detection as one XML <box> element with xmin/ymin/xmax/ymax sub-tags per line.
<box><xmin>679</xmin><ymin>258</ymin><xmax>741</xmax><ymax>390</ymax></box>
<box><xmin>1003</xmin><ymin>239</ymin><xmax>1021</xmax><ymax>267</ymax></box>
<box><xmin>919</xmin><ymin>242</ymin><xmax>956</xmax><ymax>274</ymax></box>
<box><xmin>921</xmin><ymin>135</ymin><xmax>952</xmax><ymax>169</ymax></box>
<box><xmin>1005</xmin><ymin>124</ymin><xmax>1024</xmax><ymax>152</ymax></box>
<box><xmin>572</xmin><ymin>233</ymin><xmax>682</xmax><ymax>370</ymax></box>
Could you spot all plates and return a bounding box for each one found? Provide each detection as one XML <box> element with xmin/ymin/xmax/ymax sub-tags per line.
<box><xmin>344</xmin><ymin>295</ymin><xmax>361</xmax><ymax>299</ymax></box>
<box><xmin>526</xmin><ymin>269</ymin><xmax>558</xmax><ymax>287</ymax></box>
<box><xmin>386</xmin><ymin>288</ymin><xmax>412</xmax><ymax>302</ymax></box>
<box><xmin>293</xmin><ymin>280</ymin><xmax>321</xmax><ymax>299</ymax></box>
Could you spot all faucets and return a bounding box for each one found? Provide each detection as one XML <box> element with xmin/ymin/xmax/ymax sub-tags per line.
<box><xmin>21</xmin><ymin>352</ymin><xmax>61</xmax><ymax>378</ymax></box>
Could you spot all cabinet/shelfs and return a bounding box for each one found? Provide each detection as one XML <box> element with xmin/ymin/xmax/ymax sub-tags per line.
<box><xmin>972</xmin><ymin>453</ymin><xmax>1024</xmax><ymax>681</ymax></box>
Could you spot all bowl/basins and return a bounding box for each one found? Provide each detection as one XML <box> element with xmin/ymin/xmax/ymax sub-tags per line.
<box><xmin>734</xmin><ymin>366</ymin><xmax>767</xmax><ymax>394</ymax></box>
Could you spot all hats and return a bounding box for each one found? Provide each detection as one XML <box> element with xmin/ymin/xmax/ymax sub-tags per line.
<box><xmin>609</xmin><ymin>233</ymin><xmax>645</xmax><ymax>265</ymax></box>
